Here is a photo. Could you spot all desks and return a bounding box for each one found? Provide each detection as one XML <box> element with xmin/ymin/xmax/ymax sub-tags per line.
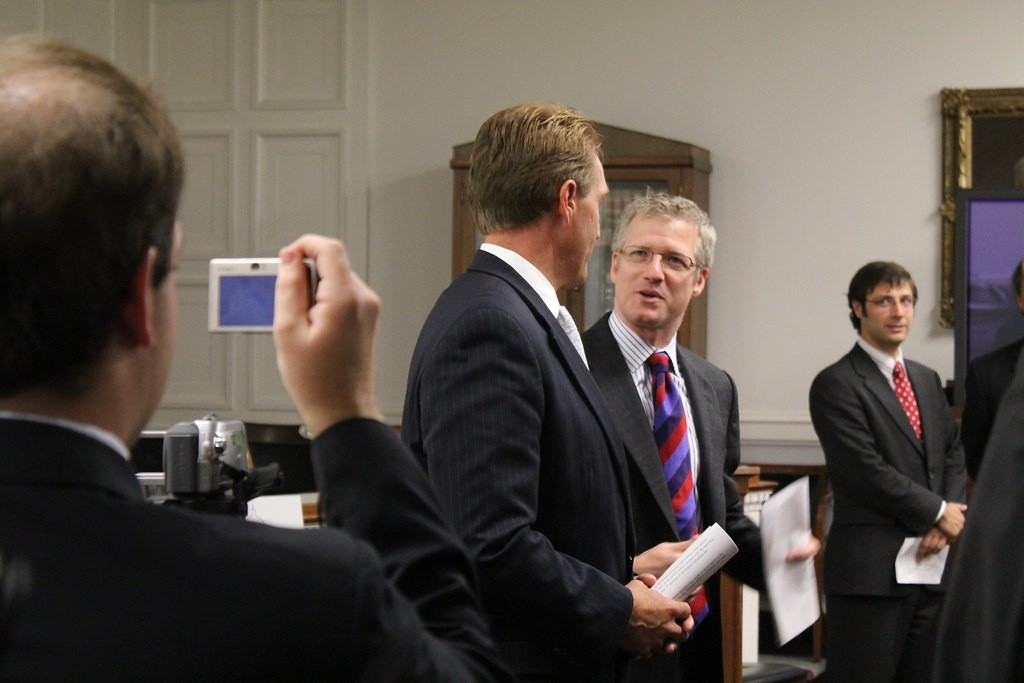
<box><xmin>738</xmin><ymin>444</ymin><xmax>835</xmax><ymax>664</ymax></box>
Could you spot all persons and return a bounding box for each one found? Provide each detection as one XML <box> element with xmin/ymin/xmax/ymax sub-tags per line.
<box><xmin>809</xmin><ymin>260</ymin><xmax>968</xmax><ymax>683</ymax></box>
<box><xmin>0</xmin><ymin>35</ymin><xmax>500</xmax><ymax>683</ymax></box>
<box><xmin>962</xmin><ymin>263</ymin><xmax>1024</xmax><ymax>683</ymax></box>
<box><xmin>402</xmin><ymin>104</ymin><xmax>694</xmax><ymax>683</ymax></box>
<box><xmin>580</xmin><ymin>184</ymin><xmax>820</xmax><ymax>683</ymax></box>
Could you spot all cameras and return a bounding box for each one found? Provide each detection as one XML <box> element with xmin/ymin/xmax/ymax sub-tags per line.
<box><xmin>208</xmin><ymin>258</ymin><xmax>320</xmax><ymax>335</ymax></box>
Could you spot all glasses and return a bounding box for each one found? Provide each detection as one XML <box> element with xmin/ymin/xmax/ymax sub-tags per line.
<box><xmin>861</xmin><ymin>297</ymin><xmax>912</xmax><ymax>308</ymax></box>
<box><xmin>621</xmin><ymin>245</ymin><xmax>699</xmax><ymax>272</ymax></box>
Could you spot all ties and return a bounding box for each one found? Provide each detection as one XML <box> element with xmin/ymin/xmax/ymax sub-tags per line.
<box><xmin>893</xmin><ymin>362</ymin><xmax>924</xmax><ymax>444</ymax></box>
<box><xmin>646</xmin><ymin>351</ymin><xmax>709</xmax><ymax>641</ymax></box>
<box><xmin>557</xmin><ymin>305</ymin><xmax>589</xmax><ymax>370</ymax></box>
